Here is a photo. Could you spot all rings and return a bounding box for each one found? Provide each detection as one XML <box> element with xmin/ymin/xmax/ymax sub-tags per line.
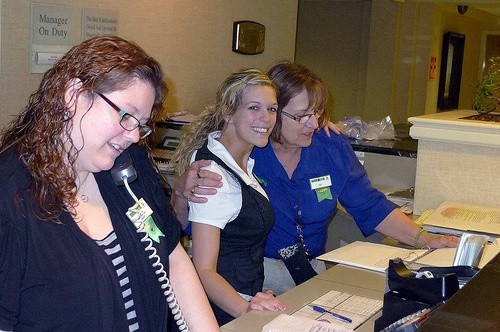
<box><xmin>197</xmin><ymin>169</ymin><xmax>202</xmax><ymax>178</ymax></box>
<box><xmin>191</xmin><ymin>186</ymin><xmax>196</xmax><ymax>195</ymax></box>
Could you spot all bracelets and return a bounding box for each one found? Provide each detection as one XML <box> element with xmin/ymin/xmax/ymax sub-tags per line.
<box><xmin>415</xmin><ymin>228</ymin><xmax>427</xmax><ymax>249</ymax></box>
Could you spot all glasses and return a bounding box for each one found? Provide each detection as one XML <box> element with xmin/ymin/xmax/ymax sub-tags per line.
<box><xmin>280</xmin><ymin>107</ymin><xmax>326</xmax><ymax>124</ymax></box>
<box><xmin>94</xmin><ymin>91</ymin><xmax>152</xmax><ymax>139</ymax></box>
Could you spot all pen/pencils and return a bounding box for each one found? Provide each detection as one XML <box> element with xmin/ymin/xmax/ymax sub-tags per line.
<box><xmin>308</xmin><ymin>304</ymin><xmax>352</xmax><ymax>323</ymax></box>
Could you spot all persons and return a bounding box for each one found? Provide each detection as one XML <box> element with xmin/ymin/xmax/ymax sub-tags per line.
<box><xmin>172</xmin><ymin>61</ymin><xmax>463</xmax><ymax>300</ymax></box>
<box><xmin>0</xmin><ymin>34</ymin><xmax>220</xmax><ymax>332</ymax></box>
<box><xmin>172</xmin><ymin>67</ymin><xmax>287</xmax><ymax>321</ymax></box>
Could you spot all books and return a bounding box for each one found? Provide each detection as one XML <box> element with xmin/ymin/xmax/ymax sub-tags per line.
<box><xmin>260</xmin><ymin>201</ymin><xmax>500</xmax><ymax>332</ymax></box>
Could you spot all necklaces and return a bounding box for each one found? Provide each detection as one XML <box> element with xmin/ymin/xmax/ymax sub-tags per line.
<box><xmin>72</xmin><ymin>171</ymin><xmax>90</xmax><ymax>202</ymax></box>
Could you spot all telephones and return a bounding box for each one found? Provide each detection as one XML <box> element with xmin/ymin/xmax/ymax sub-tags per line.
<box><xmin>111</xmin><ymin>151</ymin><xmax>137</xmax><ymax>187</ymax></box>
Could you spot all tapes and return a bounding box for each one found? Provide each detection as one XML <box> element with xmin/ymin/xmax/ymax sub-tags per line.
<box><xmin>415</xmin><ymin>271</ymin><xmax>434</xmax><ymax>279</ymax></box>
